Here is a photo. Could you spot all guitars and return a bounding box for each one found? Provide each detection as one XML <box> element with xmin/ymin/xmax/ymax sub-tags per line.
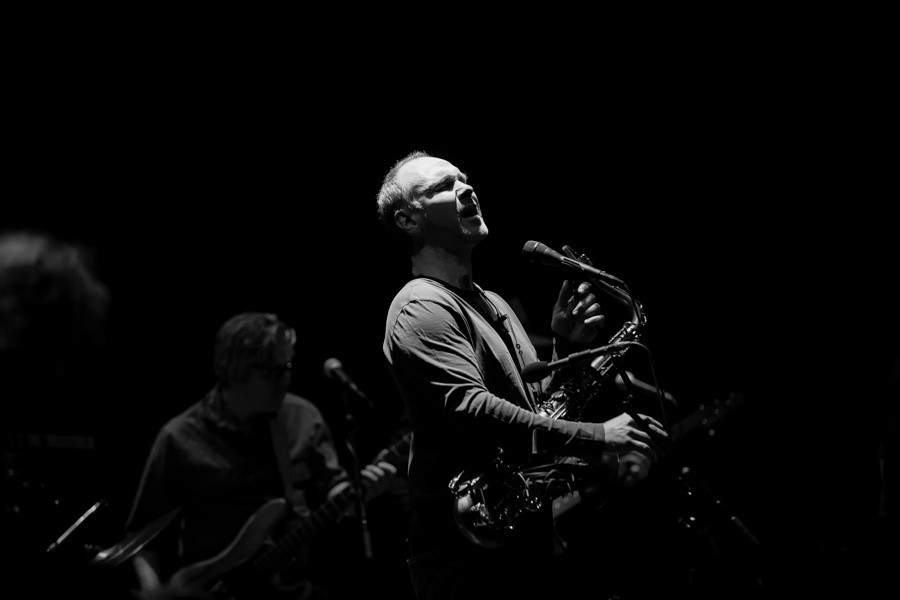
<box><xmin>593</xmin><ymin>393</ymin><xmax>744</xmax><ymax>509</ymax></box>
<box><xmin>171</xmin><ymin>432</ymin><xmax>413</xmax><ymax>600</ymax></box>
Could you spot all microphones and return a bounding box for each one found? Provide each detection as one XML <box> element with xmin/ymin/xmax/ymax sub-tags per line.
<box><xmin>324</xmin><ymin>358</ymin><xmax>360</xmax><ymax>394</ymax></box>
<box><xmin>522</xmin><ymin>341</ymin><xmax>629</xmax><ymax>384</ymax></box>
<box><xmin>522</xmin><ymin>241</ymin><xmax>625</xmax><ymax>287</ymax></box>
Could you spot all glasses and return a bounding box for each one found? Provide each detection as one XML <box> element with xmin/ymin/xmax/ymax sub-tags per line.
<box><xmin>240</xmin><ymin>362</ymin><xmax>293</xmax><ymax>380</ymax></box>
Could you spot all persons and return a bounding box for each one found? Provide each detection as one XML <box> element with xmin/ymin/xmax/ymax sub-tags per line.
<box><xmin>376</xmin><ymin>152</ymin><xmax>670</xmax><ymax>600</ymax></box>
<box><xmin>122</xmin><ymin>312</ymin><xmax>399</xmax><ymax>600</ymax></box>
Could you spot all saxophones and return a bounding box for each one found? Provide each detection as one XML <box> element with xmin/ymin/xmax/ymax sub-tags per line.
<box><xmin>448</xmin><ymin>246</ymin><xmax>648</xmax><ymax>547</ymax></box>
